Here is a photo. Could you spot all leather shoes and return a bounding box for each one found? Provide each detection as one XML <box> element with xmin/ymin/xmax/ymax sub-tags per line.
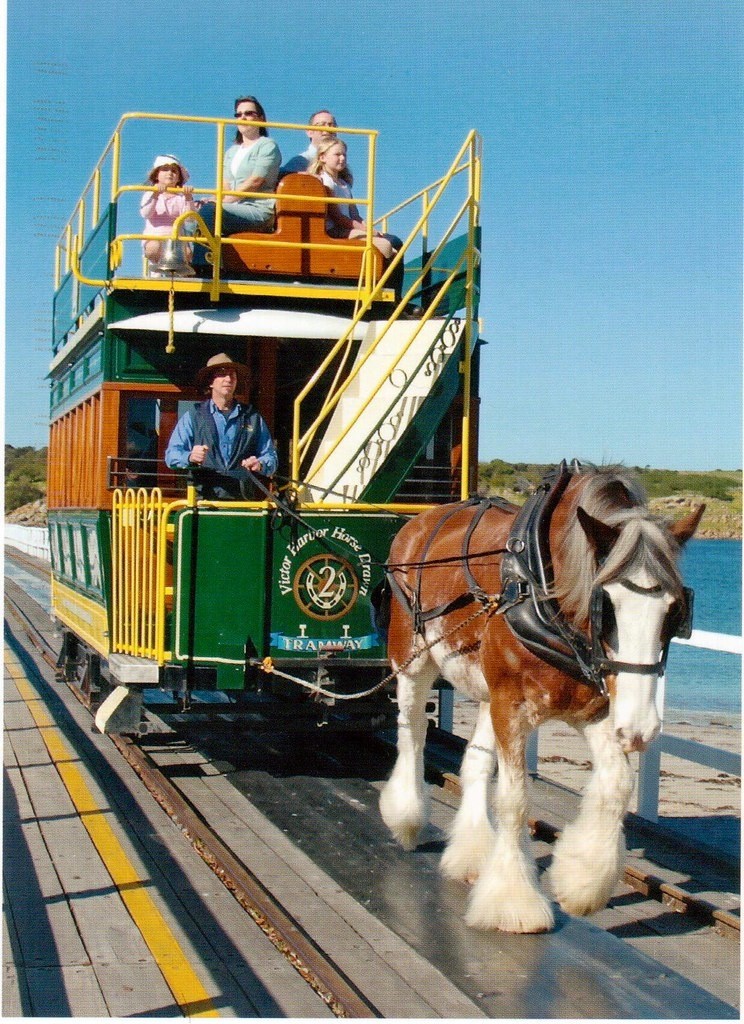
<box><xmin>396</xmin><ymin>302</ymin><xmax>424</xmax><ymax>317</ymax></box>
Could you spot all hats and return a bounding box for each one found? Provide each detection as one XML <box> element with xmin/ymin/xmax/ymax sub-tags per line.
<box><xmin>197</xmin><ymin>353</ymin><xmax>250</xmax><ymax>385</ymax></box>
<box><xmin>149</xmin><ymin>155</ymin><xmax>189</xmax><ymax>185</ymax></box>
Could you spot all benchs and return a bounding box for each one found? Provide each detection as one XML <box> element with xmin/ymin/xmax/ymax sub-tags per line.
<box><xmin>223</xmin><ymin>174</ymin><xmax>384</xmax><ymax>280</ymax></box>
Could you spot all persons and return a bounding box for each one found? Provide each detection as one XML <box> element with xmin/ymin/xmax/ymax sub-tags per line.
<box><xmin>139</xmin><ymin>154</ymin><xmax>196</xmax><ymax>277</ymax></box>
<box><xmin>279</xmin><ymin>109</ymin><xmax>424</xmax><ymax>318</ymax></box>
<box><xmin>165</xmin><ymin>353</ymin><xmax>277</xmax><ymax>501</ymax></box>
<box><xmin>196</xmin><ymin>95</ymin><xmax>282</xmax><ymax>277</ymax></box>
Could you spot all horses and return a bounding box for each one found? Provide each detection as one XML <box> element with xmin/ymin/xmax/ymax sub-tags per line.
<box><xmin>378</xmin><ymin>473</ymin><xmax>708</xmax><ymax>938</ymax></box>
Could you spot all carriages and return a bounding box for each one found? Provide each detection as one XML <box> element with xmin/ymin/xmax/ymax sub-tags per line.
<box><xmin>46</xmin><ymin>111</ymin><xmax>709</xmax><ymax>933</ymax></box>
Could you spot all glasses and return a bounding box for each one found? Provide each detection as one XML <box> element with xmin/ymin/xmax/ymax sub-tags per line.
<box><xmin>234</xmin><ymin>111</ymin><xmax>261</xmax><ymax>118</ymax></box>
<box><xmin>214</xmin><ymin>370</ymin><xmax>237</xmax><ymax>377</ymax></box>
